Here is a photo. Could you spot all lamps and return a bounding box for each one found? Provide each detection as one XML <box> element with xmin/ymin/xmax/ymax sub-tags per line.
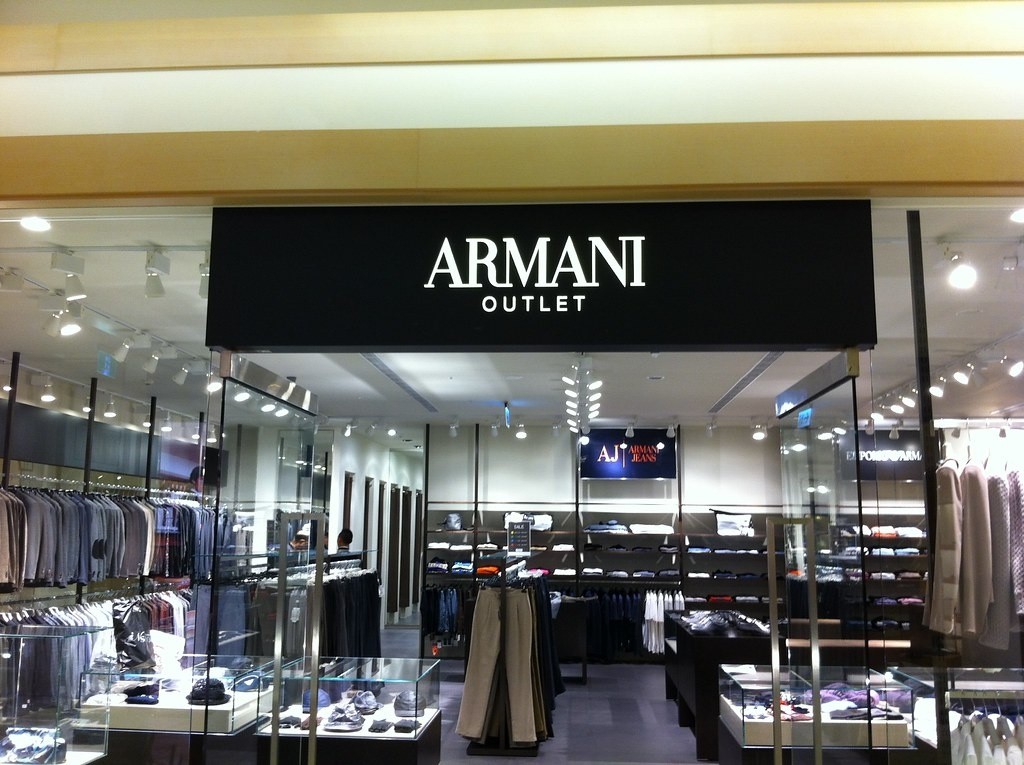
<box><xmin>1</xmin><ymin>243</ymin><xmax>1024</xmax><ymax>454</ymax></box>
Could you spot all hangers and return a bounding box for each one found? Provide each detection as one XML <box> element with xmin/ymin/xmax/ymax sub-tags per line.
<box><xmin>0</xmin><ymin>473</ymin><xmax>682</xmax><ymax>622</ymax></box>
<box><xmin>938</xmin><ymin>440</ymin><xmax>1024</xmax><ymax>728</ymax></box>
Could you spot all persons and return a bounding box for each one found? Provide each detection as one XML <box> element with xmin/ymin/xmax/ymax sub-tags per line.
<box><xmin>268</xmin><ymin>523</ymin><xmax>328</xmax><ymax>554</ymax></box>
<box><xmin>334</xmin><ymin>528</ymin><xmax>353</xmax><ymax>554</ymax></box>
<box><xmin>184</xmin><ymin>466</ymin><xmax>205</xmax><ymax>502</ymax></box>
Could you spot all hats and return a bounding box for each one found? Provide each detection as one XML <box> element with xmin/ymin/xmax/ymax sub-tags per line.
<box><xmin>186</xmin><ymin>678</ymin><xmax>231</xmax><ymax>705</ymax></box>
<box><xmin>302</xmin><ymin>688</ymin><xmax>331</xmax><ymax>713</ymax></box>
<box><xmin>394</xmin><ymin>690</ymin><xmax>427</xmax><ymax>717</ymax></box>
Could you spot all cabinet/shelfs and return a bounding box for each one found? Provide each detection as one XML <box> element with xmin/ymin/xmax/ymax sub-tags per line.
<box><xmin>421</xmin><ymin>507</ymin><xmax>938</xmax><ymax>659</ymax></box>
